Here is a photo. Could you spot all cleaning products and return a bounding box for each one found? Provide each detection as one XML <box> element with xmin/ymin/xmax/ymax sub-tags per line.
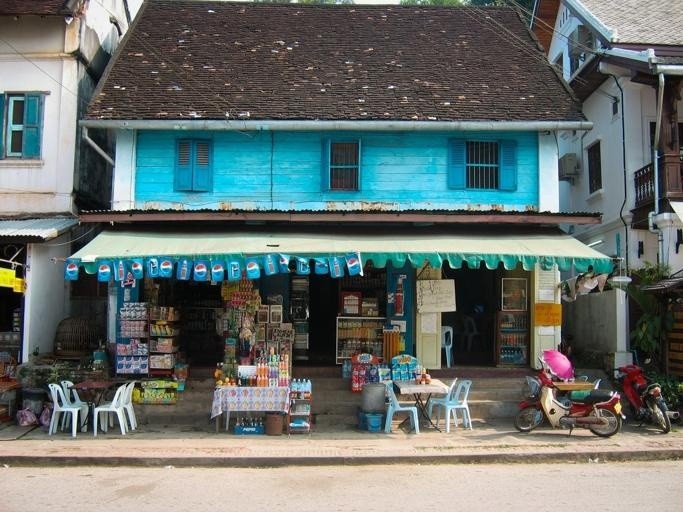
<box><xmin>312</xmin><ymin>413</ymin><xmax>321</xmax><ymax>424</ymax></box>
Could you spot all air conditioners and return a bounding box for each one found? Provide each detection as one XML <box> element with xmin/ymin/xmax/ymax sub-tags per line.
<box><xmin>568</xmin><ymin>25</ymin><xmax>592</xmax><ymax>61</ymax></box>
<box><xmin>559</xmin><ymin>153</ymin><xmax>579</xmax><ymax>185</ymax></box>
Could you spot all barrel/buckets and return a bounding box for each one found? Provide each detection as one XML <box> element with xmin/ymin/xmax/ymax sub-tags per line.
<box><xmin>357</xmin><ymin>411</ymin><xmax>368</xmax><ymax>431</ymax></box>
<box><xmin>366</xmin><ymin>413</ymin><xmax>383</xmax><ymax>433</ymax></box>
<box><xmin>290</xmin><ymin>378</ymin><xmax>312</xmax><ymax>399</ymax></box>
<box><xmin>360</xmin><ymin>383</ymin><xmax>386</xmax><ymax>413</ymax></box>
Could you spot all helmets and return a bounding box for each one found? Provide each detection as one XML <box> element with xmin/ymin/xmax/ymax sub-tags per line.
<box><xmin>522</xmin><ymin>375</ymin><xmax>542</xmax><ymax>402</ymax></box>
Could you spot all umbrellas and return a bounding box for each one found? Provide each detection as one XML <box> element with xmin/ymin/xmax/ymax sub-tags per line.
<box><xmin>538</xmin><ymin>347</ymin><xmax>576</xmax><ymax>382</ymax></box>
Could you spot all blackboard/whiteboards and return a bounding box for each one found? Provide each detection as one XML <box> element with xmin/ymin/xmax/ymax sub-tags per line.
<box><xmin>416</xmin><ymin>279</ymin><xmax>457</xmax><ymax>313</ymax></box>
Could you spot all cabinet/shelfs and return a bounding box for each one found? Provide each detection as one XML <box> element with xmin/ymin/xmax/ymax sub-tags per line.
<box><xmin>149</xmin><ymin>316</ymin><xmax>178</xmax><ymax>374</ymax></box>
<box><xmin>336</xmin><ymin>317</ymin><xmax>386</xmax><ymax>366</ymax></box>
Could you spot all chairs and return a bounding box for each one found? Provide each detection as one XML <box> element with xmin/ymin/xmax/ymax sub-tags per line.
<box><xmin>385</xmin><ymin>378</ymin><xmax>472</xmax><ymax>434</ymax></box>
<box><xmin>0</xmin><ymin>354</ymin><xmax>20</xmax><ymax>394</ymax></box>
<box><xmin>441</xmin><ymin>325</ymin><xmax>453</xmax><ymax>368</ymax></box>
<box><xmin>48</xmin><ymin>380</ymin><xmax>137</xmax><ymax>437</ymax></box>
<box><xmin>454</xmin><ymin>313</ymin><xmax>479</xmax><ymax>352</ymax></box>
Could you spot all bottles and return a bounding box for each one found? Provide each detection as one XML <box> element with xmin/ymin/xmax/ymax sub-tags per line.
<box><xmin>232</xmin><ymin>416</ymin><xmax>262</xmax><ymax>433</ymax></box>
<box><xmin>230</xmin><ymin>280</ymin><xmax>252</xmax><ymax>307</ymax></box>
<box><xmin>498</xmin><ymin>311</ymin><xmax>529</xmax><ymax>366</ymax></box>
<box><xmin>342</xmin><ymin>359</ymin><xmax>389</xmax><ymax>393</ymax></box>
<box><xmin>414</xmin><ymin>368</ymin><xmax>431</xmax><ymax>385</ymax></box>
<box><xmin>286</xmin><ymin>378</ymin><xmax>312</xmax><ymax>414</ymax></box>
<box><xmin>236</xmin><ymin>346</ymin><xmax>291</xmax><ymax>388</ymax></box>
<box><xmin>394</xmin><ymin>276</ymin><xmax>404</xmax><ymax>316</ymax></box>
<box><xmin>338</xmin><ymin>328</ymin><xmax>383</xmax><ymax>358</ymax></box>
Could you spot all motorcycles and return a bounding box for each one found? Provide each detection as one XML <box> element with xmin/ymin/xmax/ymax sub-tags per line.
<box><xmin>511</xmin><ymin>354</ymin><xmax>626</xmax><ymax>438</ymax></box>
<box><xmin>607</xmin><ymin>357</ymin><xmax>679</xmax><ymax>434</ymax></box>
